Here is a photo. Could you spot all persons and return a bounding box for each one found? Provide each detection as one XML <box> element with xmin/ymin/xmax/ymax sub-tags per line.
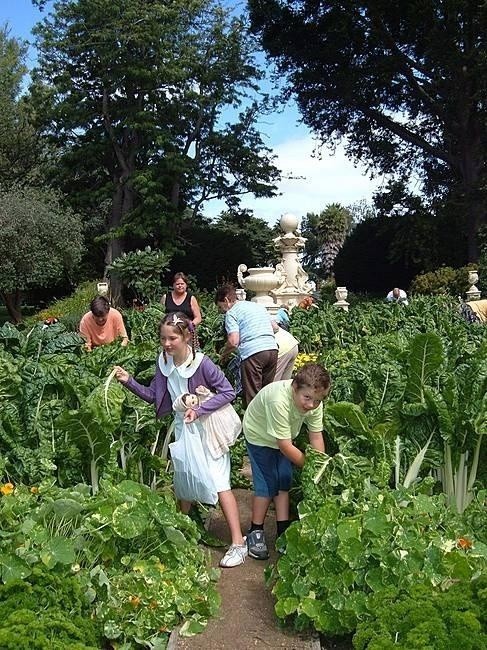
<box><xmin>384</xmin><ymin>287</ymin><xmax>407</xmax><ymax>307</ymax></box>
<box><xmin>273</xmin><ymin>303</ymin><xmax>292</xmax><ymax>332</ymax></box>
<box><xmin>156</xmin><ymin>271</ymin><xmax>202</xmax><ymax>329</ymax></box>
<box><xmin>461</xmin><ymin>299</ymin><xmax>486</xmax><ymax>325</ymax></box>
<box><xmin>271</xmin><ymin>324</ymin><xmax>301</xmax><ymax>383</ymax></box>
<box><xmin>212</xmin><ymin>283</ymin><xmax>281</xmax><ymax>412</ymax></box>
<box><xmin>109</xmin><ymin>310</ymin><xmax>250</xmax><ymax>568</ymax></box>
<box><xmin>242</xmin><ymin>360</ymin><xmax>331</xmax><ymax>561</ymax></box>
<box><xmin>133</xmin><ymin>299</ymin><xmax>145</xmax><ymax>313</ymax></box>
<box><xmin>77</xmin><ymin>293</ymin><xmax>128</xmax><ymax>353</ymax></box>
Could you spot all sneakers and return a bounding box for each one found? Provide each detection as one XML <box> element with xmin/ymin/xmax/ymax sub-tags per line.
<box><xmin>245</xmin><ymin>530</ymin><xmax>287</xmax><ymax>560</ymax></box>
<box><xmin>220</xmin><ymin>536</ymin><xmax>248</xmax><ymax>567</ymax></box>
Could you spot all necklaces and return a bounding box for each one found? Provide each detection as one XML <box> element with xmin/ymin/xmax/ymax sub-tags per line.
<box><xmin>170</xmin><ymin>356</ymin><xmax>186</xmax><ymax>367</ymax></box>
<box><xmin>174</xmin><ymin>292</ymin><xmax>181</xmax><ymax>300</ymax></box>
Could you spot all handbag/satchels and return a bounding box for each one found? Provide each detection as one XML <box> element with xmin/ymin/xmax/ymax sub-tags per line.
<box><xmin>227</xmin><ymin>355</ymin><xmax>244</xmax><ymax>396</ymax></box>
